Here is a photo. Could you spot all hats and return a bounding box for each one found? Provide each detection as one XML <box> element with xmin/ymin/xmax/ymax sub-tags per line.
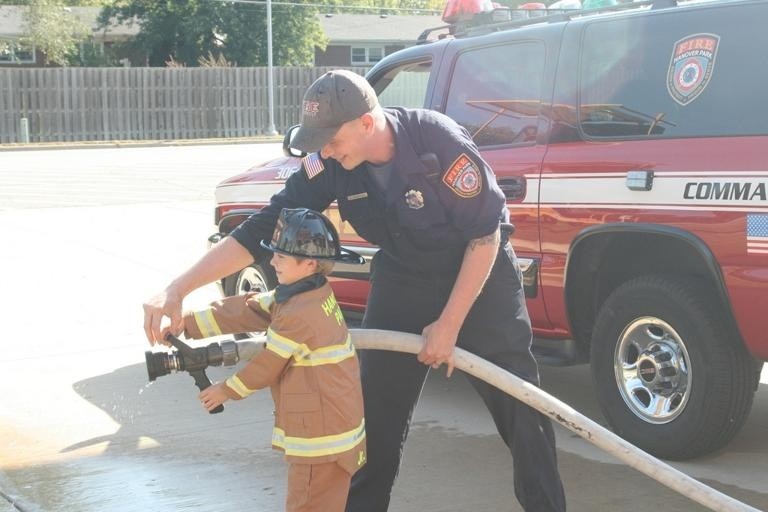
<box><xmin>287</xmin><ymin>70</ymin><xmax>378</xmax><ymax>153</ymax></box>
<box><xmin>260</xmin><ymin>208</ymin><xmax>366</xmax><ymax>265</ymax></box>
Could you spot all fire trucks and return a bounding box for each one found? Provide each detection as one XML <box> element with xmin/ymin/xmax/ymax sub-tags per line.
<box><xmin>211</xmin><ymin>0</ymin><xmax>767</xmax><ymax>465</ymax></box>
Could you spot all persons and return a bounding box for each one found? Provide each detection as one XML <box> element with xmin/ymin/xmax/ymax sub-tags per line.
<box><xmin>142</xmin><ymin>68</ymin><xmax>565</xmax><ymax>512</ymax></box>
<box><xmin>160</xmin><ymin>203</ymin><xmax>370</xmax><ymax>511</ymax></box>
